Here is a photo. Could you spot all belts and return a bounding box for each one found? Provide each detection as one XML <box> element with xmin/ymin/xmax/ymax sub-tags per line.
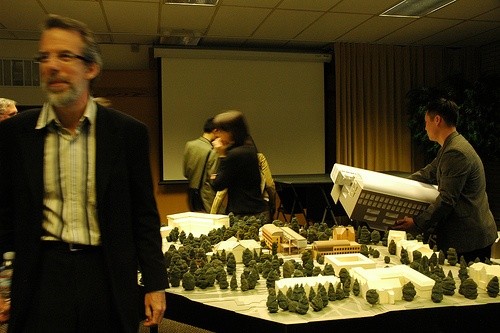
<box><xmin>30</xmin><ymin>239</ymin><xmax>105</xmax><ymax>253</ymax></box>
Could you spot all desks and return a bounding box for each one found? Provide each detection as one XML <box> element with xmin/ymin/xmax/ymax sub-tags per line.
<box><xmin>270</xmin><ymin>171</ymin><xmax>422</xmax><ymax>229</ymax></box>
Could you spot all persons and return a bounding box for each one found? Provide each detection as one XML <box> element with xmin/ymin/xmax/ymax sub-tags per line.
<box><xmin>0</xmin><ymin>14</ymin><xmax>169</xmax><ymax>333</ymax></box>
<box><xmin>209</xmin><ymin>109</ymin><xmax>281</xmax><ymax>222</ymax></box>
<box><xmin>184</xmin><ymin>117</ymin><xmax>218</xmax><ymax>213</ymax></box>
<box><xmin>391</xmin><ymin>99</ymin><xmax>498</xmax><ymax>267</ymax></box>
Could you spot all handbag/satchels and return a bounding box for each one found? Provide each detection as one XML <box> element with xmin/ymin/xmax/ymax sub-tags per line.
<box><xmin>186</xmin><ymin>187</ymin><xmax>206</xmax><ymax>212</ymax></box>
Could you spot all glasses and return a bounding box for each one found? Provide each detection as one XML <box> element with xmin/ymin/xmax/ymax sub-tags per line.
<box><xmin>33</xmin><ymin>51</ymin><xmax>91</xmax><ymax>64</ymax></box>
